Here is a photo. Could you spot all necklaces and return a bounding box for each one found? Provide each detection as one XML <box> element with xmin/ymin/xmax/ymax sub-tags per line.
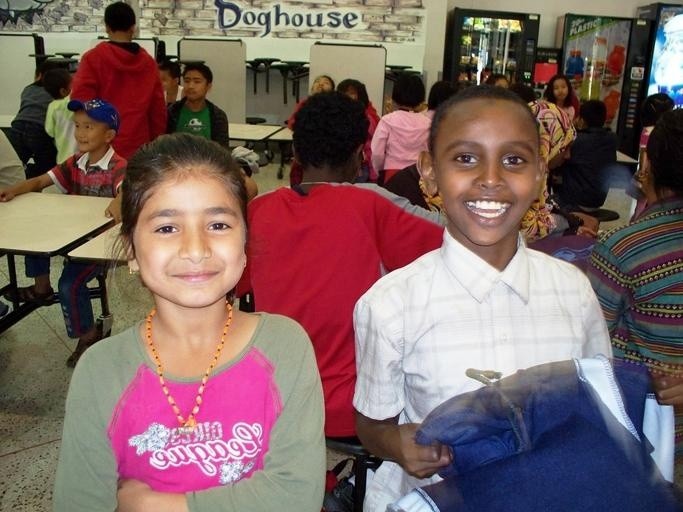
<box><xmin>146</xmin><ymin>299</ymin><xmax>231</xmax><ymax>430</ymax></box>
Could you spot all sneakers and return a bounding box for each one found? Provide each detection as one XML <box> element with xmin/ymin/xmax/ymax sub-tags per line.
<box><xmin>324</xmin><ymin>476</ymin><xmax>355</xmax><ymax>512</ymax></box>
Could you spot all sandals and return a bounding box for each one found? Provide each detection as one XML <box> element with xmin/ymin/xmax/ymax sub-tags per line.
<box><xmin>66</xmin><ymin>324</ymin><xmax>103</xmax><ymax>367</ymax></box>
<box><xmin>4</xmin><ymin>284</ymin><xmax>56</xmax><ymax>306</ymax></box>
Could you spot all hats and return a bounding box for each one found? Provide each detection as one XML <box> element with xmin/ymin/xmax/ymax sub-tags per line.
<box><xmin>67</xmin><ymin>98</ymin><xmax>119</xmax><ymax>131</ymax></box>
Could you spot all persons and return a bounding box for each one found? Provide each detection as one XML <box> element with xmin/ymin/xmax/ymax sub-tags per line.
<box><xmin>159</xmin><ymin>61</ymin><xmax>229</xmax><ymax>149</ymax></box>
<box><xmin>586</xmin><ymin>106</ymin><xmax>682</xmax><ymax>487</ymax></box>
<box><xmin>289</xmin><ymin>75</ymin><xmax>461</xmax><ymax>186</ymax></box>
<box><xmin>486</xmin><ymin>74</ymin><xmax>617</xmax><ymax>212</ymax></box>
<box><xmin>72</xmin><ymin>3</ymin><xmax>168</xmax><ymax>159</ymax></box>
<box><xmin>13</xmin><ymin>64</ymin><xmax>78</xmax><ymax>164</ymax></box>
<box><xmin>631</xmin><ymin>93</ymin><xmax>675</xmax><ymax>220</ymax></box>
<box><xmin>350</xmin><ymin>85</ymin><xmax>611</xmax><ymax>510</ymax></box>
<box><xmin>1</xmin><ymin>99</ymin><xmax>129</xmax><ymax>367</ymax></box>
<box><xmin>51</xmin><ymin>133</ymin><xmax>327</xmax><ymax>510</ymax></box>
<box><xmin>233</xmin><ymin>93</ymin><xmax>447</xmax><ymax>512</ymax></box>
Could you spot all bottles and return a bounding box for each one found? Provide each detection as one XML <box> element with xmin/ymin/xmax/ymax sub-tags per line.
<box><xmin>565</xmin><ymin>36</ymin><xmax>625</xmax><ymax>126</ymax></box>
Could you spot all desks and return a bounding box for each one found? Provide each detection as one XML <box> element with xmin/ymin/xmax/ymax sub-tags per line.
<box><xmin>0</xmin><ymin>191</ymin><xmax>118</xmax><ymax>340</ymax></box>
<box><xmin>229</xmin><ymin>123</ymin><xmax>282</xmax><ymax>141</ymax></box>
<box><xmin>269</xmin><ymin>127</ymin><xmax>294</xmax><ymax>179</ymax></box>
<box><xmin>66</xmin><ymin>221</ymin><xmax>134</xmax><ymax>267</ymax></box>
